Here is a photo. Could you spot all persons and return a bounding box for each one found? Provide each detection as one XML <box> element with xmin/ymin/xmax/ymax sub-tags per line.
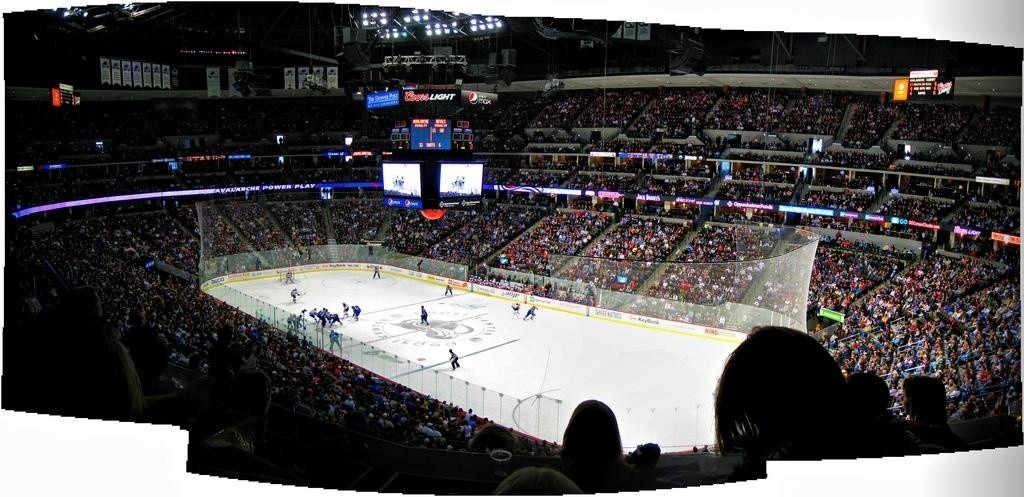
<box><xmin>6</xmin><ymin>92</ymin><xmax>1024</xmax><ymax>495</ymax></box>
